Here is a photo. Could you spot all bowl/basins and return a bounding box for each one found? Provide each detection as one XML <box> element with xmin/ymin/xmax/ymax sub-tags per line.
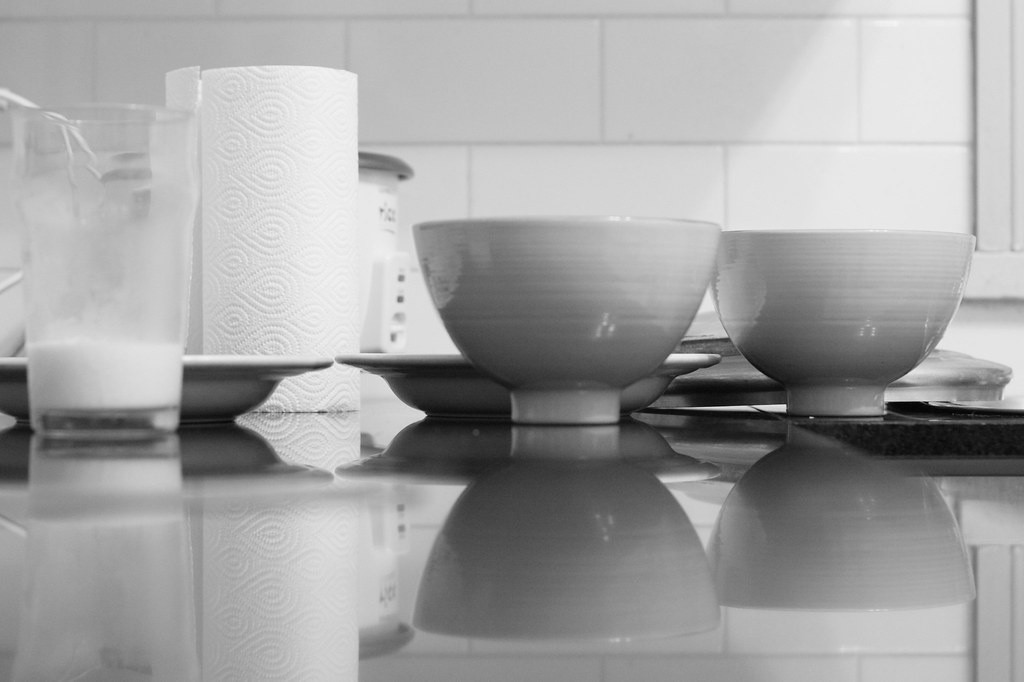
<box><xmin>413</xmin><ymin>216</ymin><xmax>722</xmax><ymax>426</ymax></box>
<box><xmin>704</xmin><ymin>424</ymin><xmax>977</xmax><ymax>613</ymax></box>
<box><xmin>709</xmin><ymin>230</ymin><xmax>976</xmax><ymax>416</ymax></box>
<box><xmin>411</xmin><ymin>424</ymin><xmax>720</xmax><ymax>646</ymax></box>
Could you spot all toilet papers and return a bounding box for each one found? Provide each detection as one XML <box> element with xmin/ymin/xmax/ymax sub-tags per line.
<box><xmin>164</xmin><ymin>64</ymin><xmax>368</xmax><ymax>411</ymax></box>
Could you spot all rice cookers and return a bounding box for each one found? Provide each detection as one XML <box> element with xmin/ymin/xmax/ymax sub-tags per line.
<box><xmin>102</xmin><ymin>147</ymin><xmax>413</xmax><ymax>353</ymax></box>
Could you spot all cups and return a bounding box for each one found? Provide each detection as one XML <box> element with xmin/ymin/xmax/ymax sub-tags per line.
<box><xmin>12</xmin><ymin>102</ymin><xmax>198</xmax><ymax>442</ymax></box>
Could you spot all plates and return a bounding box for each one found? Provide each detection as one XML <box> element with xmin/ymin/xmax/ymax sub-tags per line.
<box><xmin>332</xmin><ymin>352</ymin><xmax>722</xmax><ymax>418</ymax></box>
<box><xmin>0</xmin><ymin>353</ymin><xmax>335</xmax><ymax>427</ymax></box>
